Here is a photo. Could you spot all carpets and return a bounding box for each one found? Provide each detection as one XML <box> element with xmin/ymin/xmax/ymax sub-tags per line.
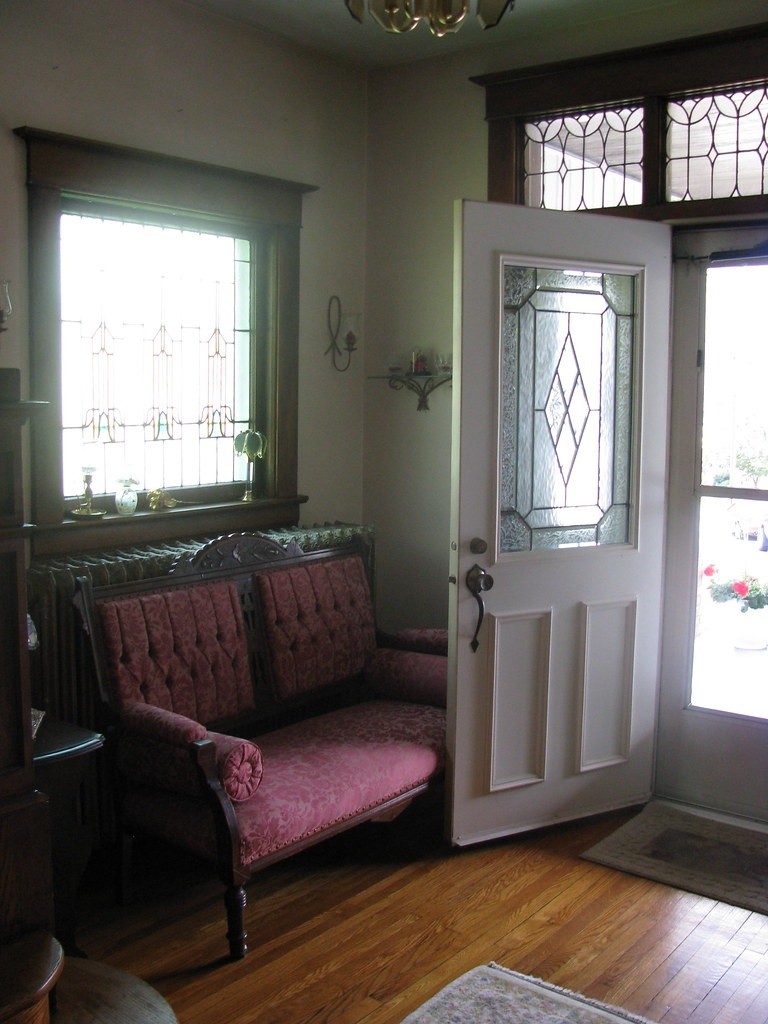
<box><xmin>398</xmin><ymin>959</ymin><xmax>657</xmax><ymax>1024</ymax></box>
<box><xmin>576</xmin><ymin>802</ymin><xmax>768</xmax><ymax>917</ymax></box>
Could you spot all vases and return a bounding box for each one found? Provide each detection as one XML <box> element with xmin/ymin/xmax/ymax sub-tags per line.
<box><xmin>723</xmin><ymin>600</ymin><xmax>768</xmax><ymax>650</ymax></box>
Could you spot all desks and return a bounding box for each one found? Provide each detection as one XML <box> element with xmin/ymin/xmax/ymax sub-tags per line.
<box><xmin>32</xmin><ymin>718</ymin><xmax>125</xmax><ymax>908</ymax></box>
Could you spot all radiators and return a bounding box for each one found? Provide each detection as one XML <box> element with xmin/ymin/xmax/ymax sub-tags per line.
<box><xmin>27</xmin><ymin>521</ymin><xmax>378</xmax><ymax>856</ymax></box>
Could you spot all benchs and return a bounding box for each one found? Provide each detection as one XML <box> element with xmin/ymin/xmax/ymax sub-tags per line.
<box><xmin>71</xmin><ymin>532</ymin><xmax>449</xmax><ymax>956</ymax></box>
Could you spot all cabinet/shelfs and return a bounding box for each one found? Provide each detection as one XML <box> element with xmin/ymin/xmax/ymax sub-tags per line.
<box><xmin>0</xmin><ymin>370</ymin><xmax>66</xmax><ymax>1024</ymax></box>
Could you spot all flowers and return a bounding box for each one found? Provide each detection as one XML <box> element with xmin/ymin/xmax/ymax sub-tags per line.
<box><xmin>704</xmin><ymin>563</ymin><xmax>768</xmax><ymax>613</ymax></box>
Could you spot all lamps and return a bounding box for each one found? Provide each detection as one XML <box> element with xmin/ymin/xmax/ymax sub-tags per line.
<box><xmin>69</xmin><ymin>466</ymin><xmax>107</xmax><ymax>520</ymax></box>
<box><xmin>0</xmin><ymin>280</ymin><xmax>14</xmax><ymax>333</ymax></box>
<box><xmin>344</xmin><ymin>0</ymin><xmax>515</xmax><ymax>37</ymax></box>
<box><xmin>324</xmin><ymin>295</ymin><xmax>357</xmax><ymax>372</ymax></box>
<box><xmin>233</xmin><ymin>428</ymin><xmax>268</xmax><ymax>502</ymax></box>
